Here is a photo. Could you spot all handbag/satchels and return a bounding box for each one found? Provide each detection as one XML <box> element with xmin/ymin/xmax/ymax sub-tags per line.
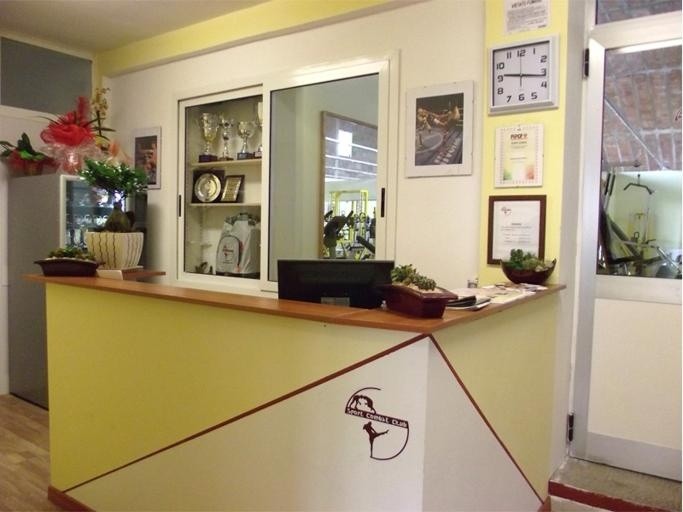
<box><xmin>216</xmin><ymin>212</ymin><xmax>260</xmax><ymax>278</ymax></box>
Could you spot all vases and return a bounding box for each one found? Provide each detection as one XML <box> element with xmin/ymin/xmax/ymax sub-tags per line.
<box><xmin>81</xmin><ymin>232</ymin><xmax>143</xmax><ymax>272</ymax></box>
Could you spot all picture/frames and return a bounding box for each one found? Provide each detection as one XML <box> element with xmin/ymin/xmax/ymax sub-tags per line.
<box><xmin>129</xmin><ymin>127</ymin><xmax>163</xmax><ymax>190</ymax></box>
<box><xmin>401</xmin><ymin>81</ymin><xmax>477</xmax><ymax>180</ymax></box>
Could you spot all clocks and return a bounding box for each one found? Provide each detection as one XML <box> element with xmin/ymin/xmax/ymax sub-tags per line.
<box><xmin>485</xmin><ymin>35</ymin><xmax>559</xmax><ymax>117</ymax></box>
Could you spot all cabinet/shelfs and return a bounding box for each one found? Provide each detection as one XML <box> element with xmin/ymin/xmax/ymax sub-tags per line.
<box><xmin>177</xmin><ymin>84</ymin><xmax>266</xmax><ymax>287</ymax></box>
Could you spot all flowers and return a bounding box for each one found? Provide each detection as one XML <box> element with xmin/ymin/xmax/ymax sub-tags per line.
<box><xmin>37</xmin><ymin>87</ymin><xmax>151</xmax><ymax>232</ymax></box>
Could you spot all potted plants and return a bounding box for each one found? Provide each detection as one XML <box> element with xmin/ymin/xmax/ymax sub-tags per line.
<box><xmin>34</xmin><ymin>242</ymin><xmax>107</xmax><ymax>277</ymax></box>
<box><xmin>498</xmin><ymin>247</ymin><xmax>559</xmax><ymax>284</ymax></box>
<box><xmin>387</xmin><ymin>262</ymin><xmax>459</xmax><ymax>321</ymax></box>
